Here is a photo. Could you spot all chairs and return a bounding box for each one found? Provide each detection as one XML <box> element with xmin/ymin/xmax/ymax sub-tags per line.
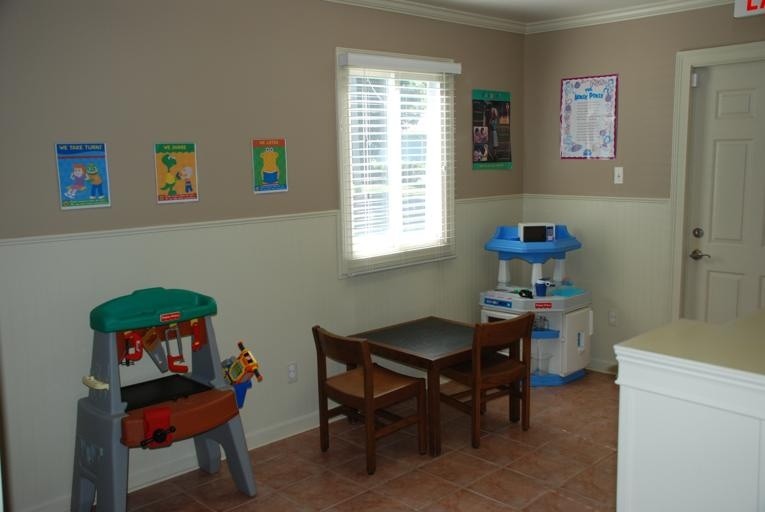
<box><xmin>312</xmin><ymin>324</ymin><xmax>427</xmax><ymax>475</ymax></box>
<box><xmin>441</xmin><ymin>313</ymin><xmax>535</xmax><ymax>449</ymax></box>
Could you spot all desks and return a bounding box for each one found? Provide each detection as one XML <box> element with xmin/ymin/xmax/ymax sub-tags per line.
<box><xmin>344</xmin><ymin>315</ymin><xmax>521</xmax><ymax>456</ymax></box>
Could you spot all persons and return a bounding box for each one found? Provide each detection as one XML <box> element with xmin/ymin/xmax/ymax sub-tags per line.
<box><xmin>473</xmin><ymin>108</ymin><xmax>499</xmax><ymax>163</ymax></box>
